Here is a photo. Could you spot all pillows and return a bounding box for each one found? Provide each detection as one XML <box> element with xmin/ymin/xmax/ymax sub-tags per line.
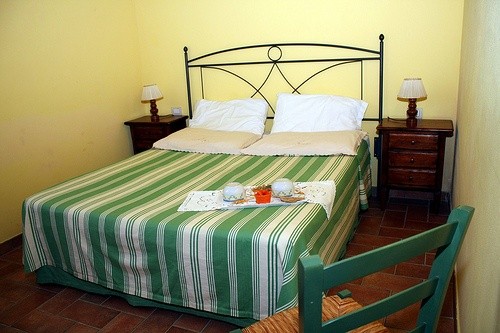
<box><xmin>242</xmin><ymin>130</ymin><xmax>368</xmax><ymax>157</ymax></box>
<box><xmin>187</xmin><ymin>99</ymin><xmax>268</xmax><ymax>137</ymax></box>
<box><xmin>270</xmin><ymin>93</ymin><xmax>369</xmax><ymax>131</ymax></box>
<box><xmin>152</xmin><ymin>127</ymin><xmax>263</xmax><ymax>156</ymax></box>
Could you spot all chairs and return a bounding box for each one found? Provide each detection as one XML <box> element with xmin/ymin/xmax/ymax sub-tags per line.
<box><xmin>227</xmin><ymin>204</ymin><xmax>475</xmax><ymax>333</ymax></box>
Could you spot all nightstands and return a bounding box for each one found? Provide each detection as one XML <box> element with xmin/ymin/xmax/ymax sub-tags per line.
<box><xmin>123</xmin><ymin>116</ymin><xmax>188</xmax><ymax>154</ymax></box>
<box><xmin>376</xmin><ymin>118</ymin><xmax>454</xmax><ymax>208</ymax></box>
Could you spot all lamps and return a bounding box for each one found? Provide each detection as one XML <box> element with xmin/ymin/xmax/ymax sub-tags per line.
<box><xmin>140</xmin><ymin>84</ymin><xmax>164</xmax><ymax>119</ymax></box>
<box><xmin>397</xmin><ymin>77</ymin><xmax>428</xmax><ymax>123</ymax></box>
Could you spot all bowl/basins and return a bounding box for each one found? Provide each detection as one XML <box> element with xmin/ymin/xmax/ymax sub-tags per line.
<box><xmin>271</xmin><ymin>178</ymin><xmax>293</xmax><ymax>197</ymax></box>
<box><xmin>223</xmin><ymin>182</ymin><xmax>246</xmax><ymax>202</ymax></box>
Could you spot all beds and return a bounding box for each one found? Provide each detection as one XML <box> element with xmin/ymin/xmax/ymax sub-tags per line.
<box><xmin>21</xmin><ymin>33</ymin><xmax>384</xmax><ymax>328</ymax></box>
